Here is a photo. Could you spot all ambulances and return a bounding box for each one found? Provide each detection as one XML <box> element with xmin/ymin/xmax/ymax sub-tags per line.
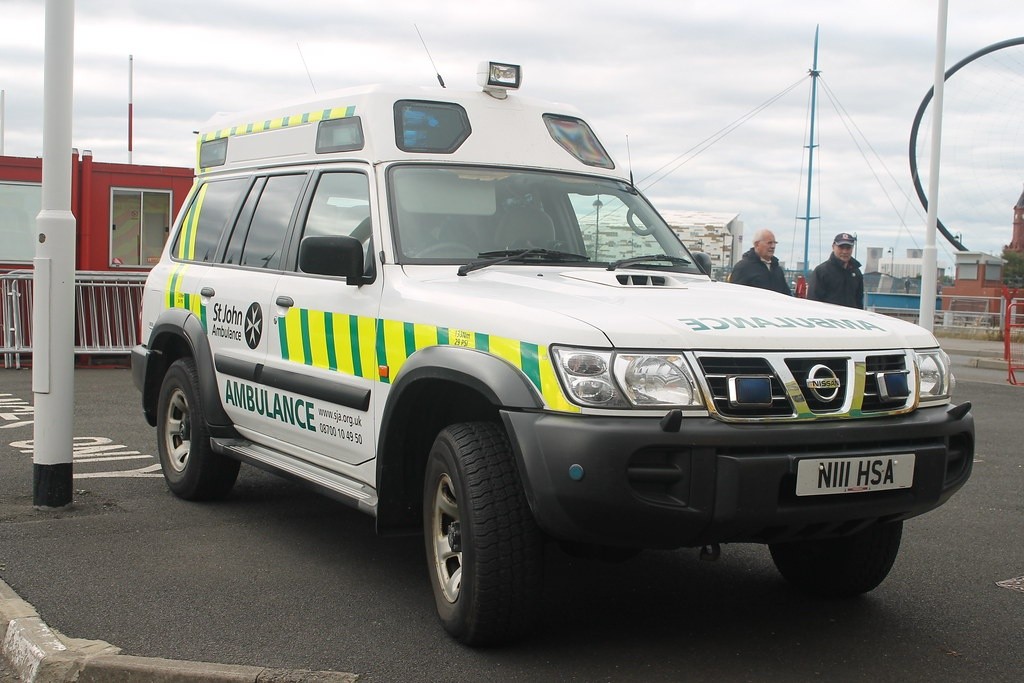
<box><xmin>129</xmin><ymin>60</ymin><xmax>975</xmax><ymax>646</ymax></box>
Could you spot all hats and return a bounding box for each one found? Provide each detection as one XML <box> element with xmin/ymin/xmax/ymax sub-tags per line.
<box><xmin>834</xmin><ymin>233</ymin><xmax>854</xmax><ymax>247</ymax></box>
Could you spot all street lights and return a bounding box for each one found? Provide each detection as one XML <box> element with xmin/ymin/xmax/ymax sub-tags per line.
<box><xmin>954</xmin><ymin>231</ymin><xmax>962</xmax><ymax>246</ymax></box>
<box><xmin>888</xmin><ymin>247</ymin><xmax>894</xmax><ymax>278</ymax></box>
<box><xmin>593</xmin><ymin>194</ymin><xmax>602</xmax><ymax>261</ymax></box>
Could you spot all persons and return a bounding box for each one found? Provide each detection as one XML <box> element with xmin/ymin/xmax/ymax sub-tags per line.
<box><xmin>729</xmin><ymin>228</ymin><xmax>792</xmax><ymax>296</ymax></box>
<box><xmin>905</xmin><ymin>276</ymin><xmax>911</xmax><ymax>294</ymax></box>
<box><xmin>807</xmin><ymin>233</ymin><xmax>864</xmax><ymax>310</ymax></box>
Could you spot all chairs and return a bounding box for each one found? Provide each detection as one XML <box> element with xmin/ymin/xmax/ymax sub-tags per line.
<box><xmin>496</xmin><ymin>205</ymin><xmax>555</xmax><ymax>250</ymax></box>
